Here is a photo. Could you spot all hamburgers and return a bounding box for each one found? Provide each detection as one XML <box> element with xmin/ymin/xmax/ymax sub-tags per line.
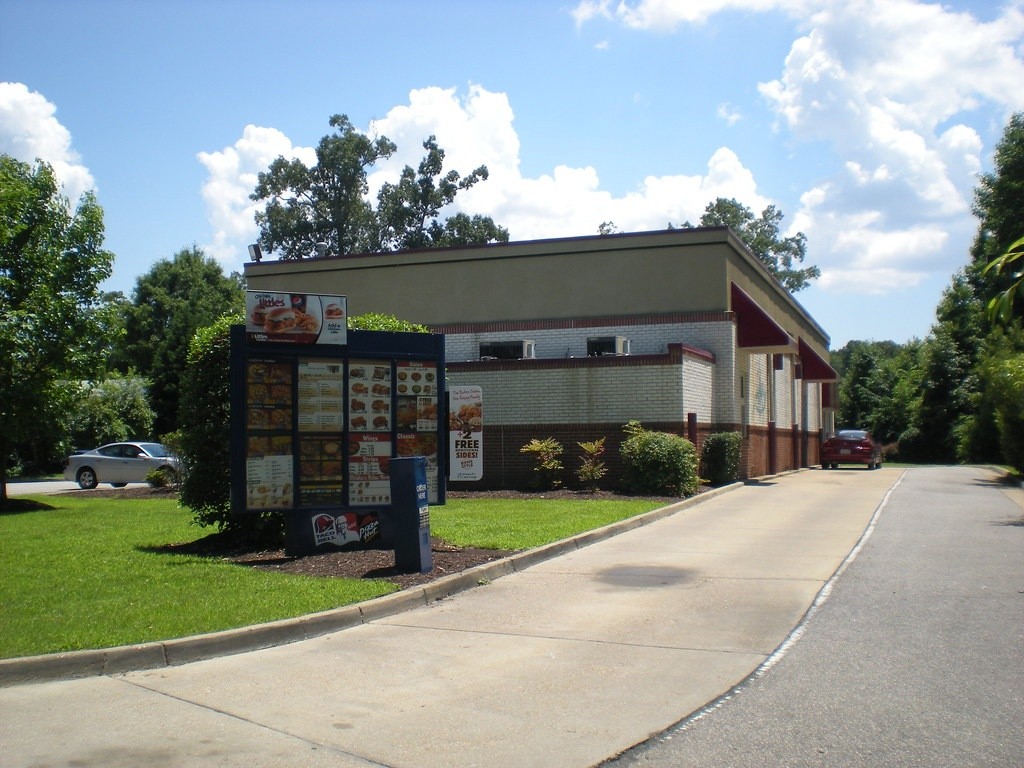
<box><xmin>325</xmin><ymin>303</ymin><xmax>343</xmax><ymax>321</ymax></box>
<box><xmin>250</xmin><ymin>304</ymin><xmax>298</xmax><ymax>333</ymax></box>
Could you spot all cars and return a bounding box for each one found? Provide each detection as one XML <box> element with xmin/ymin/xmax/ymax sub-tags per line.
<box><xmin>818</xmin><ymin>429</ymin><xmax>884</xmax><ymax>470</ymax></box>
<box><xmin>62</xmin><ymin>441</ymin><xmax>185</xmax><ymax>490</ymax></box>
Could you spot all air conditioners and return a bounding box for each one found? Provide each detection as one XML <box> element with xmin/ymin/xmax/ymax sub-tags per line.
<box><xmin>479</xmin><ymin>339</ymin><xmax>535</xmax><ymax>359</ymax></box>
<box><xmin>587</xmin><ymin>336</ymin><xmax>628</xmax><ymax>357</ymax></box>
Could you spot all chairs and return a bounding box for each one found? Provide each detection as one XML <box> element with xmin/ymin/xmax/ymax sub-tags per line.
<box><xmin>130</xmin><ymin>448</ymin><xmax>142</xmax><ymax>458</ymax></box>
